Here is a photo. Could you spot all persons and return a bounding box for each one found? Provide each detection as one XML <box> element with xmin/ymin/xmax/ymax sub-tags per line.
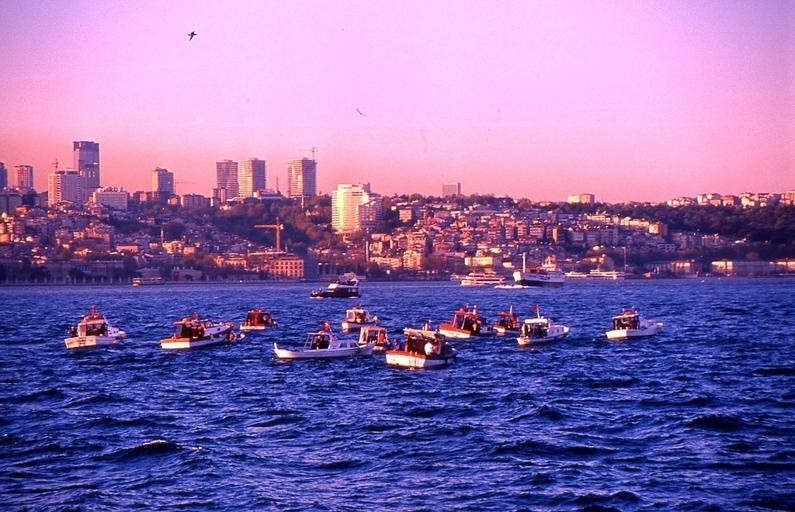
<box><xmin>424</xmin><ymin>337</ymin><xmax>434</xmax><ymax>358</ymax></box>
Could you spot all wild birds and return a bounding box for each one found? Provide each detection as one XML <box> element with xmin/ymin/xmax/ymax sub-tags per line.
<box><xmin>355</xmin><ymin>108</ymin><xmax>368</xmax><ymax>118</ymax></box>
<box><xmin>187</xmin><ymin>30</ymin><xmax>198</xmax><ymax>41</ymax></box>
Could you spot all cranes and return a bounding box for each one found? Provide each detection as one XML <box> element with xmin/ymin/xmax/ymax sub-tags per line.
<box><xmin>249</xmin><ymin>216</ymin><xmax>288</xmax><ymax>250</ymax></box>
<box><xmin>49</xmin><ymin>158</ymin><xmax>59</xmax><ymax>169</ymax></box>
<box><xmin>299</xmin><ymin>145</ymin><xmax>320</xmax><ymax>160</ymax></box>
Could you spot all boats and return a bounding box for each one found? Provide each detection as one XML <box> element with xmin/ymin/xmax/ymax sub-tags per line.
<box><xmin>239</xmin><ymin>304</ymin><xmax>278</xmax><ymax>333</ymax></box>
<box><xmin>512</xmin><ymin>303</ymin><xmax>572</xmax><ymax>349</ymax></box>
<box><xmin>63</xmin><ymin>306</ymin><xmax>127</xmax><ymax>353</ymax></box>
<box><xmin>493</xmin><ymin>303</ymin><xmax>525</xmax><ymax>337</ymax></box>
<box><xmin>338</xmin><ymin>304</ymin><xmax>380</xmax><ymax>333</ymax></box>
<box><xmin>453</xmin><ymin>268</ymin><xmax>511</xmax><ymax>291</ymax></box>
<box><xmin>510</xmin><ymin>251</ymin><xmax>640</xmax><ymax>289</ymax></box>
<box><xmin>355</xmin><ymin>323</ymin><xmax>394</xmax><ymax>354</ymax></box>
<box><xmin>437</xmin><ymin>302</ymin><xmax>499</xmax><ymax>341</ymax></box>
<box><xmin>269</xmin><ymin>318</ymin><xmax>380</xmax><ymax>362</ymax></box>
<box><xmin>131</xmin><ymin>277</ymin><xmax>166</xmax><ymax>287</ymax></box>
<box><xmin>605</xmin><ymin>305</ymin><xmax>664</xmax><ymax>343</ymax></box>
<box><xmin>156</xmin><ymin>311</ymin><xmax>238</xmax><ymax>353</ymax></box>
<box><xmin>303</xmin><ymin>270</ymin><xmax>368</xmax><ymax>302</ymax></box>
<box><xmin>385</xmin><ymin>317</ymin><xmax>460</xmax><ymax>370</ymax></box>
<box><xmin>447</xmin><ymin>272</ymin><xmax>466</xmax><ymax>282</ymax></box>
